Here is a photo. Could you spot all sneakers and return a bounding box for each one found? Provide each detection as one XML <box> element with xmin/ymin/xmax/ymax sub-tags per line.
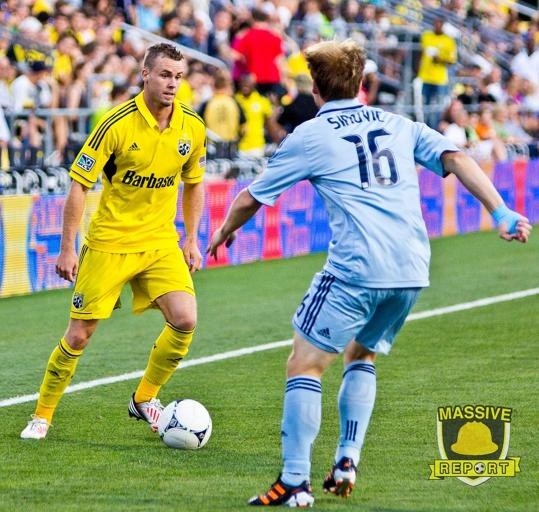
<box><xmin>20</xmin><ymin>418</ymin><xmax>51</xmax><ymax>439</ymax></box>
<box><xmin>248</xmin><ymin>472</ymin><xmax>315</xmax><ymax>507</ymax></box>
<box><xmin>322</xmin><ymin>457</ymin><xmax>358</xmax><ymax>497</ymax></box>
<box><xmin>128</xmin><ymin>392</ymin><xmax>163</xmax><ymax>431</ymax></box>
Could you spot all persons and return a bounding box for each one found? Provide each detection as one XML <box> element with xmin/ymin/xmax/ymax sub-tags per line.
<box><xmin>199</xmin><ymin>39</ymin><xmax>535</xmax><ymax>507</ymax></box>
<box><xmin>0</xmin><ymin>1</ymin><xmax>539</xmax><ymax>176</ymax></box>
<box><xmin>17</xmin><ymin>43</ymin><xmax>207</xmax><ymax>440</ymax></box>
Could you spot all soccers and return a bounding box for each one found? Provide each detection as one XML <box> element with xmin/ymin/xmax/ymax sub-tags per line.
<box><xmin>157</xmin><ymin>399</ymin><xmax>212</xmax><ymax>450</ymax></box>
